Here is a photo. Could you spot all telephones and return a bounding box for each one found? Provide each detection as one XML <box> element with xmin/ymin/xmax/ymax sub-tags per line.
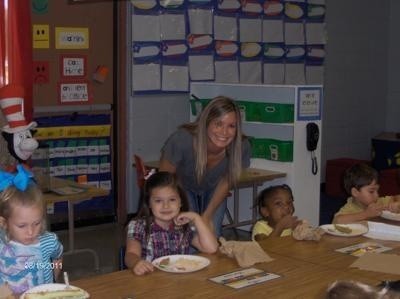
<box><xmin>307</xmin><ymin>123</ymin><xmax>319</xmax><ymax>151</ymax></box>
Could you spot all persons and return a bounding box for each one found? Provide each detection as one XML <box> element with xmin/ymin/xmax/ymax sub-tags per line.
<box><xmin>331</xmin><ymin>162</ymin><xmax>400</xmax><ymax>224</ymax></box>
<box><xmin>159</xmin><ymin>96</ymin><xmax>252</xmax><ymax>257</ymax></box>
<box><xmin>1</xmin><ymin>165</ymin><xmax>64</xmax><ymax>299</ymax></box>
<box><xmin>123</xmin><ymin>167</ymin><xmax>219</xmax><ymax>275</ymax></box>
<box><xmin>251</xmin><ymin>184</ymin><xmax>307</xmax><ymax>243</ymax></box>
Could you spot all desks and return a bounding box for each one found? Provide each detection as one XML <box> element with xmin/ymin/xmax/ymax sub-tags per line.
<box><xmin>132</xmin><ymin>160</ymin><xmax>288</xmax><ymax>241</ymax></box>
<box><xmin>32</xmin><ymin>176</ymin><xmax>108</xmax><ymax>273</ymax></box>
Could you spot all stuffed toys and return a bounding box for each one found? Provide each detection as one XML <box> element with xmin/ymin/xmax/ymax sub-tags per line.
<box><xmin>0</xmin><ymin>85</ymin><xmax>40</xmax><ymax>175</ymax></box>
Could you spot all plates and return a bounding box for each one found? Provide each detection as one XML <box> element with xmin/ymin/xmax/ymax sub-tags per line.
<box><xmin>19</xmin><ymin>283</ymin><xmax>90</xmax><ymax>299</ymax></box>
<box><xmin>152</xmin><ymin>255</ymin><xmax>210</xmax><ymax>273</ymax></box>
<box><xmin>320</xmin><ymin>224</ymin><xmax>369</xmax><ymax>236</ymax></box>
